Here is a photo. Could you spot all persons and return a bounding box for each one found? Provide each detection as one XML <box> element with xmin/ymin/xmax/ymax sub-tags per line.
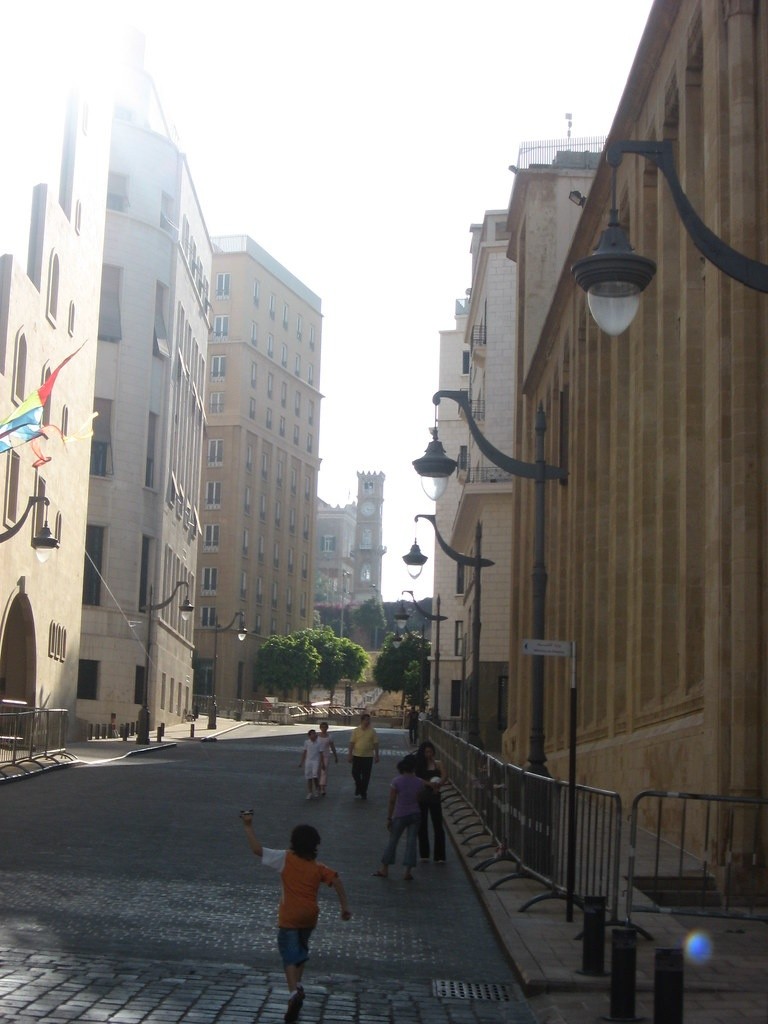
<box><xmin>405</xmin><ymin>706</ymin><xmax>419</xmax><ymax>745</ymax></box>
<box><xmin>238</xmin><ymin>809</ymin><xmax>353</xmax><ymax>1024</ymax></box>
<box><xmin>348</xmin><ymin>714</ymin><xmax>380</xmax><ymax>800</ymax></box>
<box><xmin>368</xmin><ymin>741</ymin><xmax>447</xmax><ymax>881</ymax></box>
<box><xmin>301</xmin><ymin>722</ymin><xmax>339</xmax><ymax>800</ymax></box>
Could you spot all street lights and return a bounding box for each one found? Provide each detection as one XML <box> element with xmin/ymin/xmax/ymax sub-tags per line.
<box><xmin>135</xmin><ymin>578</ymin><xmax>196</xmax><ymax>747</ymax></box>
<box><xmin>205</xmin><ymin>610</ymin><xmax>248</xmax><ymax>731</ymax></box>
<box><xmin>406</xmin><ymin>388</ymin><xmax>570</xmax><ymax>881</ymax></box>
<box><xmin>392</xmin><ymin>588</ymin><xmax>450</xmax><ymax>729</ymax></box>
<box><xmin>392</xmin><ymin>621</ymin><xmax>432</xmax><ymax>719</ymax></box>
<box><xmin>399</xmin><ymin>511</ymin><xmax>495</xmax><ymax>750</ymax></box>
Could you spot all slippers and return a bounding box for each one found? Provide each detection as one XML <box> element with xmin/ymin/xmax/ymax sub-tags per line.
<box><xmin>370</xmin><ymin>871</ymin><xmax>384</xmax><ymax>876</ymax></box>
<box><xmin>405</xmin><ymin>876</ymin><xmax>413</xmax><ymax>881</ymax></box>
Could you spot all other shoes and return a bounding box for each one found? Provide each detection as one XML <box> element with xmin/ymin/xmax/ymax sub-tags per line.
<box><xmin>314</xmin><ymin>792</ymin><xmax>320</xmax><ymax>799</ymax></box>
<box><xmin>321</xmin><ymin>790</ymin><xmax>326</xmax><ymax>795</ymax></box>
<box><xmin>354</xmin><ymin>787</ymin><xmax>362</xmax><ymax>796</ymax></box>
<box><xmin>439</xmin><ymin>859</ymin><xmax>446</xmax><ymax>864</ymax></box>
<box><xmin>306</xmin><ymin>793</ymin><xmax>312</xmax><ymax>800</ymax></box>
<box><xmin>284</xmin><ymin>992</ymin><xmax>303</xmax><ymax>1022</ymax></box>
<box><xmin>361</xmin><ymin>791</ymin><xmax>368</xmax><ymax>800</ymax></box>
<box><xmin>298</xmin><ymin>986</ymin><xmax>305</xmax><ymax>998</ymax></box>
<box><xmin>421</xmin><ymin>857</ymin><xmax>429</xmax><ymax>862</ymax></box>
